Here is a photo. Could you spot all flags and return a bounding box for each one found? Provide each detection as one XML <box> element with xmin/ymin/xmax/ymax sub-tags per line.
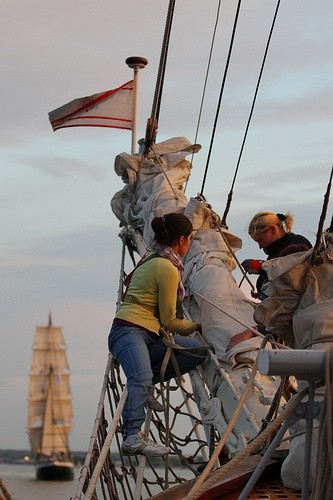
<box><xmin>48</xmin><ymin>80</ymin><xmax>135</xmax><ymax>132</ymax></box>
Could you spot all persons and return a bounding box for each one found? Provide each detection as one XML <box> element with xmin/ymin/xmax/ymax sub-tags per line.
<box><xmin>224</xmin><ymin>211</ymin><xmax>313</xmax><ymax>353</ymax></box>
<box><xmin>107</xmin><ymin>211</ymin><xmax>208</xmax><ymax>456</ymax></box>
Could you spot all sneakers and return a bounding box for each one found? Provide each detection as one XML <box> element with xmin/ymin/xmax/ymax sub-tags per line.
<box><xmin>122</xmin><ymin>432</ymin><xmax>169</xmax><ymax>456</ymax></box>
<box><xmin>146</xmin><ymin>391</ymin><xmax>165</xmax><ymax>412</ymax></box>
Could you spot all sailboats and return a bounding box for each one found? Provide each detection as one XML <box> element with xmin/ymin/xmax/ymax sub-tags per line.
<box><xmin>69</xmin><ymin>2</ymin><xmax>333</xmax><ymax>500</ymax></box>
<box><xmin>23</xmin><ymin>307</ymin><xmax>75</xmax><ymax>482</ymax></box>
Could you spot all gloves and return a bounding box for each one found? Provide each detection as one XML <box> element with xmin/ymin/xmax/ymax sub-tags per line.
<box><xmin>241</xmin><ymin>259</ymin><xmax>264</xmax><ymax>274</ymax></box>
